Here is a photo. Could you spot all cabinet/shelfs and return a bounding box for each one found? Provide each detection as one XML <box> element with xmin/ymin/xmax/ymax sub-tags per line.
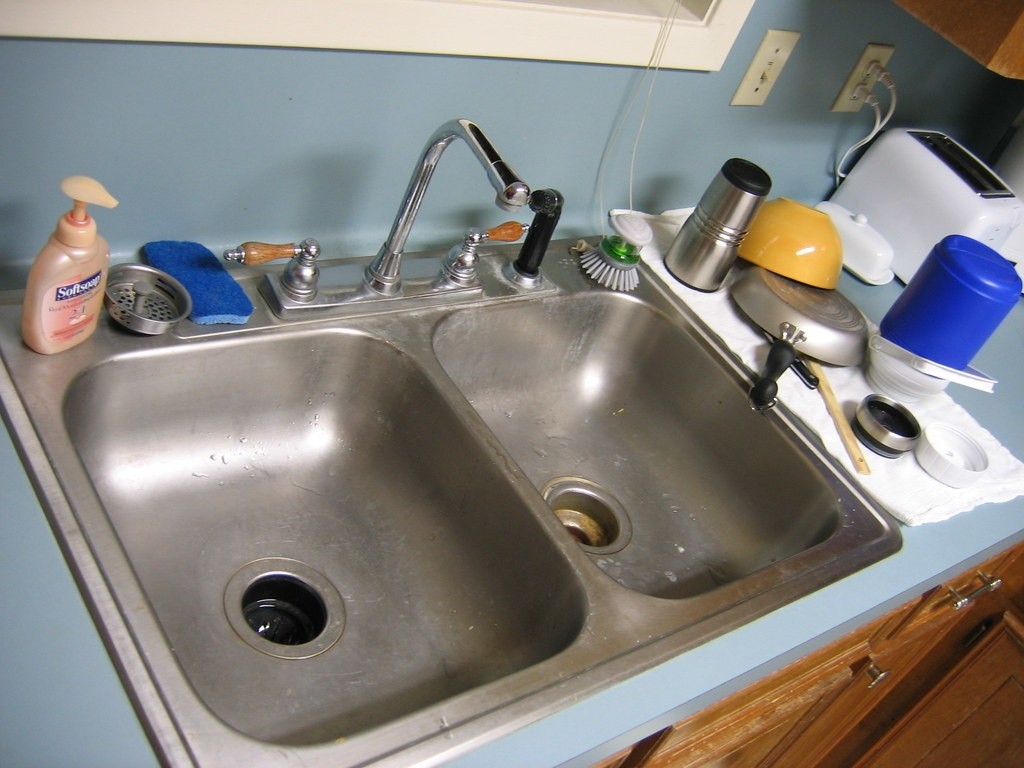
<box><xmin>586</xmin><ymin>541</ymin><xmax>1024</xmax><ymax>768</ymax></box>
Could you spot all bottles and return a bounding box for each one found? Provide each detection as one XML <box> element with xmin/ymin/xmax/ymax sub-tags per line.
<box><xmin>663</xmin><ymin>158</ymin><xmax>773</xmax><ymax>293</ymax></box>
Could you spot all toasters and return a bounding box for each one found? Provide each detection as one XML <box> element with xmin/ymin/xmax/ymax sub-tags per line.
<box><xmin>826</xmin><ymin>127</ymin><xmax>1024</xmax><ymax>287</ymax></box>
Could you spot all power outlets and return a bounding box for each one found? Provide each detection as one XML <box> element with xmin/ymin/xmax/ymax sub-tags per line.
<box><xmin>830</xmin><ymin>43</ymin><xmax>896</xmax><ymax>114</ymax></box>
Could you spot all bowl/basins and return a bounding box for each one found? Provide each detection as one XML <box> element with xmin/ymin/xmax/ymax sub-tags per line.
<box><xmin>735</xmin><ymin>197</ymin><xmax>843</xmax><ymax>290</ymax></box>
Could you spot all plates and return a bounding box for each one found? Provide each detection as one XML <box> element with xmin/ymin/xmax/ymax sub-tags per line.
<box><xmin>730</xmin><ymin>266</ymin><xmax>868</xmax><ymax>367</ymax></box>
